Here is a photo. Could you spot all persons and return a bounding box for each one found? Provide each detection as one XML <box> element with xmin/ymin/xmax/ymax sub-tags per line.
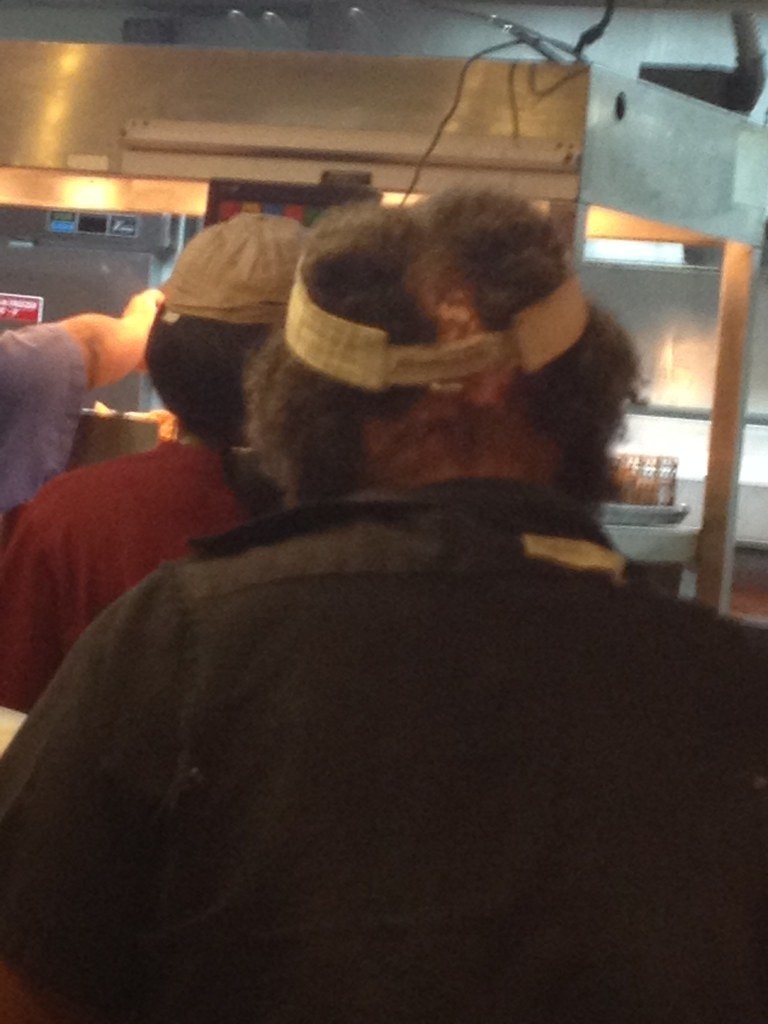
<box><xmin>0</xmin><ymin>188</ymin><xmax>767</xmax><ymax>1024</ymax></box>
<box><xmin>0</xmin><ymin>213</ymin><xmax>312</xmax><ymax>752</ymax></box>
<box><xmin>0</xmin><ymin>287</ymin><xmax>166</xmax><ymax>515</ymax></box>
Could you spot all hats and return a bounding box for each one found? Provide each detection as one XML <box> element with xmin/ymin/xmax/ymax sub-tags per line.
<box><xmin>160</xmin><ymin>203</ymin><xmax>313</xmax><ymax>325</ymax></box>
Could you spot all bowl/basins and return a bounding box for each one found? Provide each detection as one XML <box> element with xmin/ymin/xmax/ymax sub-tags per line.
<box><xmin>602</xmin><ymin>524</ymin><xmax>703</xmax><ymax>562</ymax></box>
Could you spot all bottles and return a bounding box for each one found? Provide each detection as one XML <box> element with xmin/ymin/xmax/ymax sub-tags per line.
<box><xmin>607</xmin><ymin>453</ymin><xmax>678</xmax><ymax>506</ymax></box>
